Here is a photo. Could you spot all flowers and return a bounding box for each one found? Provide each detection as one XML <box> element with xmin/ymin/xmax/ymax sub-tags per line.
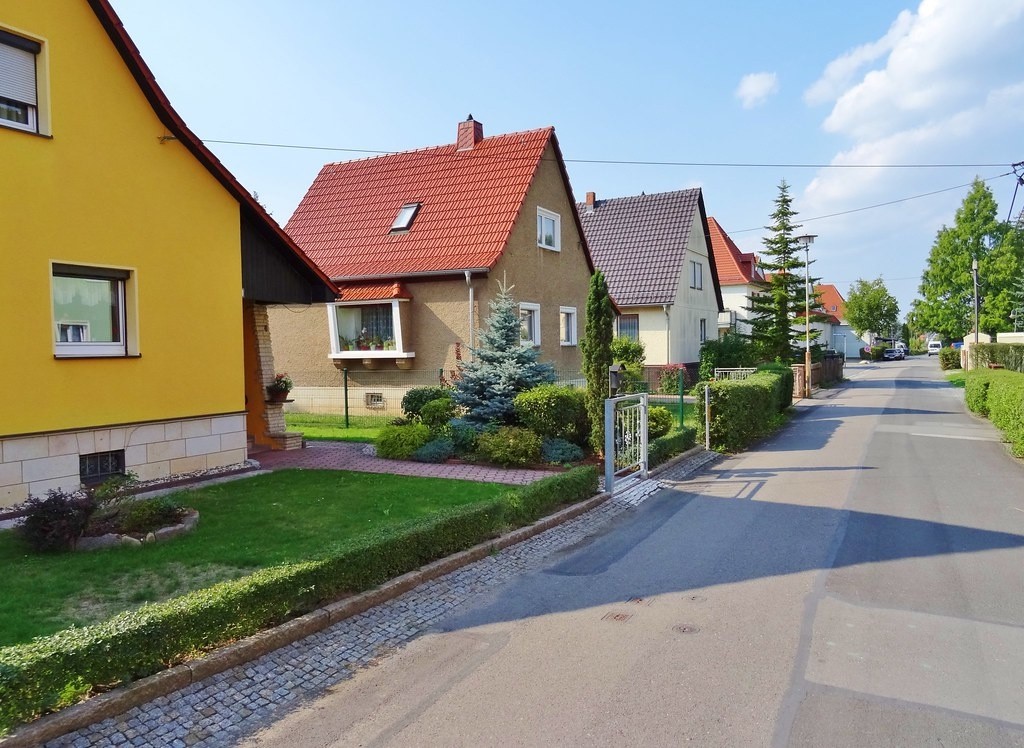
<box><xmin>266</xmin><ymin>372</ymin><xmax>293</xmax><ymax>394</ymax></box>
<box><xmin>360</xmin><ymin>327</ymin><xmax>368</xmax><ymax>346</ymax></box>
<box><xmin>384</xmin><ymin>336</ymin><xmax>393</xmax><ymax>350</ymax></box>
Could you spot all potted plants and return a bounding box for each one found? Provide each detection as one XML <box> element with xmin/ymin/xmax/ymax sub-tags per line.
<box><xmin>368</xmin><ymin>336</ymin><xmax>382</xmax><ymax>351</ymax></box>
<box><xmin>339</xmin><ymin>334</ymin><xmax>359</xmax><ymax>350</ymax></box>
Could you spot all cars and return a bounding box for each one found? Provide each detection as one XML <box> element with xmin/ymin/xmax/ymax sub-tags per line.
<box><xmin>882</xmin><ymin>347</ymin><xmax>904</xmax><ymax>361</ymax></box>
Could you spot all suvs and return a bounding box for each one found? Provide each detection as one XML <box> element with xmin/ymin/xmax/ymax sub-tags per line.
<box><xmin>928</xmin><ymin>340</ymin><xmax>943</xmax><ymax>356</ymax></box>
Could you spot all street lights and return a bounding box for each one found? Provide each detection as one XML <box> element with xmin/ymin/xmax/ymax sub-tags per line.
<box><xmin>972</xmin><ymin>253</ymin><xmax>978</xmax><ymax>345</ymax></box>
<box><xmin>797</xmin><ymin>234</ymin><xmax>819</xmax><ymax>398</ymax></box>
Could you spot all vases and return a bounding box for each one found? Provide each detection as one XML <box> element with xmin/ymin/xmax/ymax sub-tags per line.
<box><xmin>389</xmin><ymin>346</ymin><xmax>394</xmax><ymax>350</ymax></box>
<box><xmin>272</xmin><ymin>392</ymin><xmax>289</xmax><ymax>401</ymax></box>
<box><xmin>361</xmin><ymin>346</ymin><xmax>368</xmax><ymax>350</ymax></box>
